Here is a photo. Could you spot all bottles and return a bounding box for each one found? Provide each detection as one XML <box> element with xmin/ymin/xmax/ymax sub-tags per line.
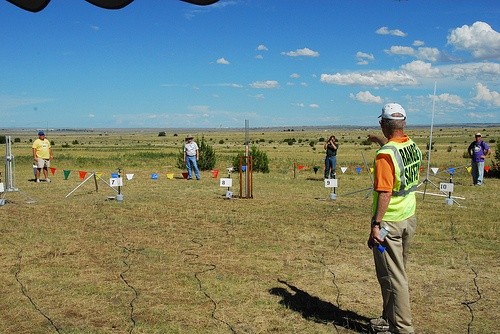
<box><xmin>368</xmin><ymin>226</ymin><xmax>390</xmax><ymax>249</ymax></box>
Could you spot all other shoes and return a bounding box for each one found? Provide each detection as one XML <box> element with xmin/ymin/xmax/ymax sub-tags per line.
<box><xmin>45</xmin><ymin>178</ymin><xmax>51</xmax><ymax>182</ymax></box>
<box><xmin>197</xmin><ymin>178</ymin><xmax>200</xmax><ymax>180</ymax></box>
<box><xmin>36</xmin><ymin>179</ymin><xmax>40</xmax><ymax>182</ymax></box>
<box><xmin>187</xmin><ymin>178</ymin><xmax>192</xmax><ymax>180</ymax></box>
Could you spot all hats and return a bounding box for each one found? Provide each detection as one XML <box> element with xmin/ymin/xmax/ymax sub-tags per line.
<box><xmin>475</xmin><ymin>132</ymin><xmax>482</xmax><ymax>137</ymax></box>
<box><xmin>38</xmin><ymin>131</ymin><xmax>44</xmax><ymax>135</ymax></box>
<box><xmin>378</xmin><ymin>103</ymin><xmax>406</xmax><ymax>120</ymax></box>
<box><xmin>185</xmin><ymin>135</ymin><xmax>194</xmax><ymax>139</ymax></box>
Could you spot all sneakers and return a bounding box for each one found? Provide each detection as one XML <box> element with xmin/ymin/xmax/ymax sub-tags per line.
<box><xmin>377</xmin><ymin>330</ymin><xmax>395</xmax><ymax>334</ymax></box>
<box><xmin>370</xmin><ymin>316</ymin><xmax>392</xmax><ymax>328</ymax></box>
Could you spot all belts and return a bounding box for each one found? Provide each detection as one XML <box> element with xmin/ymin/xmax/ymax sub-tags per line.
<box><xmin>186</xmin><ymin>155</ymin><xmax>196</xmax><ymax>157</ymax></box>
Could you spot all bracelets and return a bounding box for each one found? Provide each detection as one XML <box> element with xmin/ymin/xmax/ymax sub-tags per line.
<box><xmin>373</xmin><ymin>219</ymin><xmax>380</xmax><ymax>225</ymax></box>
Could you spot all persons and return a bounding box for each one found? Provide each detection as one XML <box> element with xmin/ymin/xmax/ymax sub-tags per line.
<box><xmin>366</xmin><ymin>102</ymin><xmax>422</xmax><ymax>334</ymax></box>
<box><xmin>32</xmin><ymin>131</ymin><xmax>53</xmax><ymax>182</ymax></box>
<box><xmin>324</xmin><ymin>136</ymin><xmax>338</xmax><ymax>180</ymax></box>
<box><xmin>183</xmin><ymin>134</ymin><xmax>201</xmax><ymax>180</ymax></box>
<box><xmin>467</xmin><ymin>133</ymin><xmax>491</xmax><ymax>186</ymax></box>
